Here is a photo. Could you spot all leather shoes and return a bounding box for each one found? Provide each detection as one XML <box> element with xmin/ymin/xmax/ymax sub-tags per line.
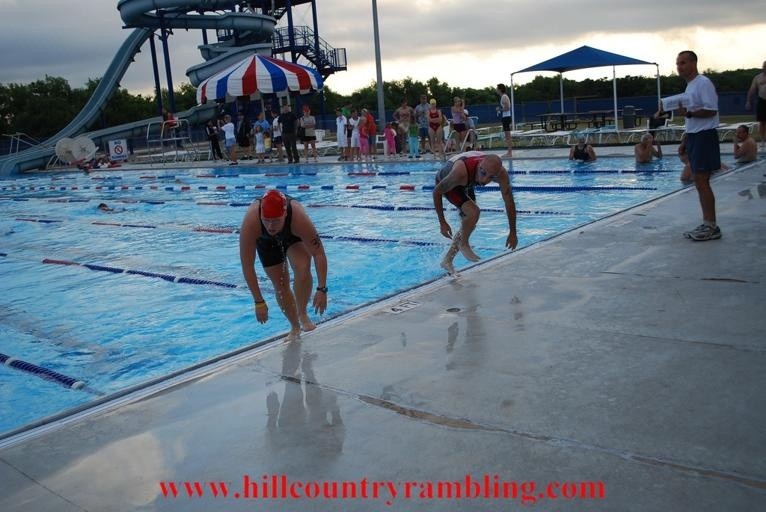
<box><xmin>257</xmin><ymin>159</ymin><xmax>265</xmax><ymax>164</ymax></box>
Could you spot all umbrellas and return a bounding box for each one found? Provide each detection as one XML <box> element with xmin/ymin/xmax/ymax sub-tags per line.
<box><xmin>196</xmin><ymin>48</ymin><xmax>324</xmax><ymax>119</ymax></box>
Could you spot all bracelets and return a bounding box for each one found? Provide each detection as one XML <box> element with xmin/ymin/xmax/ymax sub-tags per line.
<box><xmin>254</xmin><ymin>299</ymin><xmax>265</xmax><ymax>304</ymax></box>
<box><xmin>238</xmin><ymin>189</ymin><xmax>327</xmax><ymax>343</ymax></box>
<box><xmin>686</xmin><ymin>110</ymin><xmax>692</xmax><ymax>118</ymax></box>
<box><xmin>255</xmin><ymin>302</ymin><xmax>266</xmax><ymax>308</ymax></box>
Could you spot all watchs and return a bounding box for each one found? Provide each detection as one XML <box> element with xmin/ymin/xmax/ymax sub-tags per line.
<box><xmin>316</xmin><ymin>287</ymin><xmax>328</xmax><ymax>293</ymax></box>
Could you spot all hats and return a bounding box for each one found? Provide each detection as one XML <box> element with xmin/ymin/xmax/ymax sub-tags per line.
<box><xmin>336</xmin><ymin>107</ymin><xmax>341</xmax><ymax>111</ymax></box>
<box><xmin>454</xmin><ymin>97</ymin><xmax>460</xmax><ymax>102</ymax></box>
<box><xmin>464</xmin><ymin>109</ymin><xmax>469</xmax><ymax>116</ymax></box>
<box><xmin>303</xmin><ymin>105</ymin><xmax>310</xmax><ymax>113</ymax></box>
<box><xmin>430</xmin><ymin>99</ymin><xmax>437</xmax><ymax>104</ymax></box>
<box><xmin>261</xmin><ymin>190</ymin><xmax>288</xmax><ymax>218</ymax></box>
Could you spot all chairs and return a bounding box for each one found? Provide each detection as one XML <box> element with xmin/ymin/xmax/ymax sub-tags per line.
<box><xmin>163</xmin><ymin>105</ymin><xmax>764</xmax><ymax>160</ymax></box>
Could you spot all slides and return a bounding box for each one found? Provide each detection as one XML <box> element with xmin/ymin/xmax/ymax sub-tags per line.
<box><xmin>0</xmin><ymin>0</ymin><xmax>278</xmax><ymax>176</ymax></box>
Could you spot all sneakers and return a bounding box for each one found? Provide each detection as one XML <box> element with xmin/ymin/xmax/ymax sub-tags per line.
<box><xmin>229</xmin><ymin>162</ymin><xmax>239</xmax><ymax>165</ymax></box>
<box><xmin>338</xmin><ymin>156</ymin><xmax>351</xmax><ymax>161</ymax></box>
<box><xmin>249</xmin><ymin>155</ymin><xmax>253</xmax><ymax>160</ymax></box>
<box><xmin>239</xmin><ymin>155</ymin><xmax>249</xmax><ymax>160</ymax></box>
<box><xmin>683</xmin><ymin>224</ymin><xmax>722</xmax><ymax>242</ymax></box>
<box><xmin>466</xmin><ymin>145</ymin><xmax>472</xmax><ymax>152</ymax></box>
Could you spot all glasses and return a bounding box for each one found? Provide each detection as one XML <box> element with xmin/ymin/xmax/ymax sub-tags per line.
<box><xmin>478</xmin><ymin>164</ymin><xmax>498</xmax><ymax>182</ymax></box>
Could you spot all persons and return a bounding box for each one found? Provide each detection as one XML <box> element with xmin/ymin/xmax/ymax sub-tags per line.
<box><xmin>634</xmin><ymin>133</ymin><xmax>663</xmax><ymax>163</ymax></box>
<box><xmin>76</xmin><ymin>157</ymin><xmax>112</xmax><ymax>170</ymax></box>
<box><xmin>746</xmin><ymin>61</ymin><xmax>766</xmax><ymax>143</ymax></box>
<box><xmin>676</xmin><ymin>49</ymin><xmax>722</xmax><ymax>242</ymax></box>
<box><xmin>678</xmin><ymin>131</ymin><xmax>728</xmax><ymax>175</ymax></box>
<box><xmin>203</xmin><ymin>93</ymin><xmax>478</xmax><ymax>165</ymax></box>
<box><xmin>496</xmin><ymin>83</ymin><xmax>513</xmax><ymax>158</ymax></box>
<box><xmin>161</xmin><ymin>106</ymin><xmax>177</xmax><ymax>127</ymax></box>
<box><xmin>648</xmin><ymin>101</ymin><xmax>672</xmax><ymax>139</ymax></box>
<box><xmin>569</xmin><ymin>136</ymin><xmax>597</xmax><ymax>160</ymax></box>
<box><xmin>733</xmin><ymin>125</ymin><xmax>757</xmax><ymax>163</ymax></box>
<box><xmin>432</xmin><ymin>151</ymin><xmax>519</xmax><ymax>278</ymax></box>
<box><xmin>97</xmin><ymin>203</ymin><xmax>114</xmax><ymax>211</ymax></box>
